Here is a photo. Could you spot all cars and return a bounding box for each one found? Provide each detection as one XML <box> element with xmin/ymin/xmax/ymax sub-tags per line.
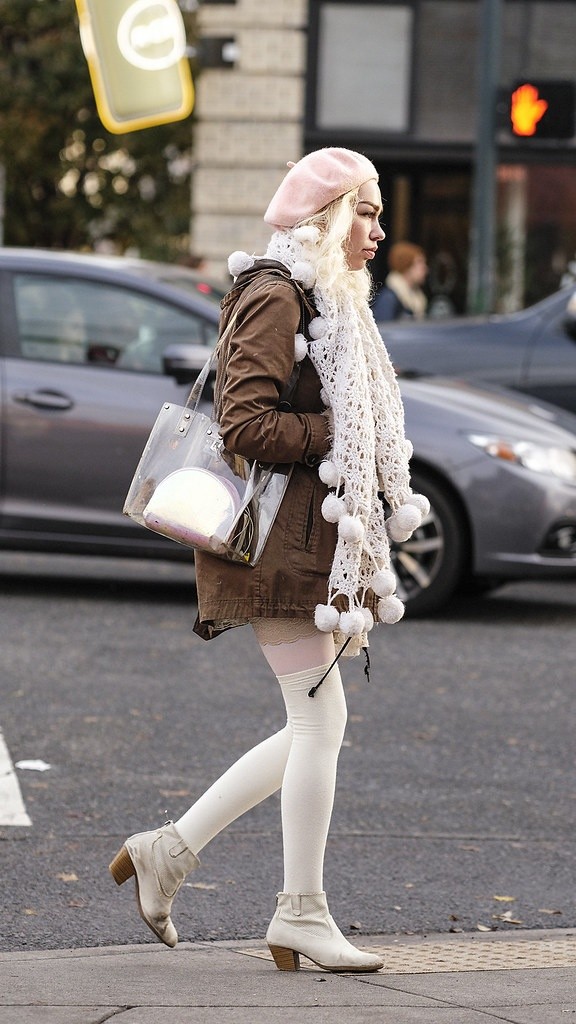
<box><xmin>372</xmin><ymin>278</ymin><xmax>575</xmax><ymax>418</ymax></box>
<box><xmin>1</xmin><ymin>246</ymin><xmax>575</xmax><ymax>618</ymax></box>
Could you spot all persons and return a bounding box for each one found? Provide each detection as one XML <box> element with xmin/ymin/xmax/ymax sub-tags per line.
<box><xmin>370</xmin><ymin>240</ymin><xmax>428</xmax><ymax>325</ymax></box>
<box><xmin>108</xmin><ymin>148</ymin><xmax>433</xmax><ymax>978</ymax></box>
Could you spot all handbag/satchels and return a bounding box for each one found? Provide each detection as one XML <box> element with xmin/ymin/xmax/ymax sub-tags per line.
<box><xmin>142</xmin><ymin>467</ymin><xmax>241</xmax><ymax>556</ymax></box>
<box><xmin>122</xmin><ymin>280</ymin><xmax>306</xmax><ymax>567</ymax></box>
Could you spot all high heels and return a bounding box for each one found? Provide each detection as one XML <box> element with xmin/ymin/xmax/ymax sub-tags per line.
<box><xmin>265</xmin><ymin>892</ymin><xmax>384</xmax><ymax>972</ymax></box>
<box><xmin>109</xmin><ymin>819</ymin><xmax>200</xmax><ymax>948</ymax></box>
<box><xmin>145</xmin><ymin>850</ymin><xmax>147</xmax><ymax>853</ymax></box>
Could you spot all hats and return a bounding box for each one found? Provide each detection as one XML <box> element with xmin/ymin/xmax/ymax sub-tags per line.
<box><xmin>390</xmin><ymin>242</ymin><xmax>422</xmax><ymax>270</ymax></box>
<box><xmin>264</xmin><ymin>146</ymin><xmax>379</xmax><ymax>232</ymax></box>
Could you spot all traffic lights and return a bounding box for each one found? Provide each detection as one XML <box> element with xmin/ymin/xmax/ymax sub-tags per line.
<box><xmin>506</xmin><ymin>82</ymin><xmax>576</xmax><ymax>143</ymax></box>
